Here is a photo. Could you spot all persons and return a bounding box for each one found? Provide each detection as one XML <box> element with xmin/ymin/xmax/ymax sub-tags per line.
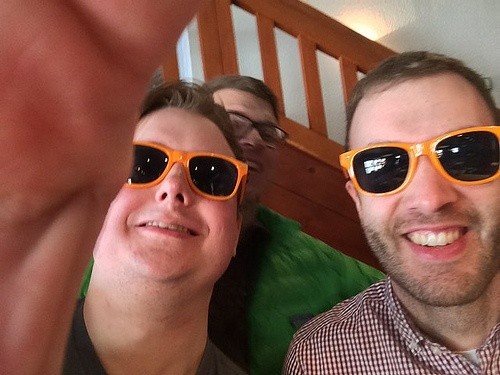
<box><xmin>281</xmin><ymin>50</ymin><xmax>500</xmax><ymax>375</ymax></box>
<box><xmin>0</xmin><ymin>0</ymin><xmax>248</xmax><ymax>375</ymax></box>
<box><xmin>79</xmin><ymin>74</ymin><xmax>388</xmax><ymax>375</ymax></box>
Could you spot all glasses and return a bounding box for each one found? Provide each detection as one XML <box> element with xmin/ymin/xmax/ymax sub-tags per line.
<box><xmin>338</xmin><ymin>126</ymin><xmax>500</xmax><ymax>197</ymax></box>
<box><xmin>123</xmin><ymin>140</ymin><xmax>248</xmax><ymax>210</ymax></box>
<box><xmin>226</xmin><ymin>111</ymin><xmax>289</xmax><ymax>151</ymax></box>
